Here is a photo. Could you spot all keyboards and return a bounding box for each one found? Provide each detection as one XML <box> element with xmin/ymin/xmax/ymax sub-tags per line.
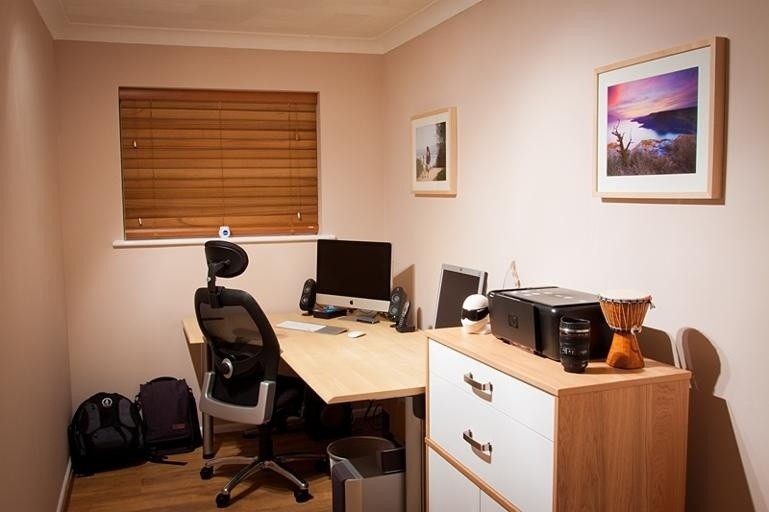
<box><xmin>275</xmin><ymin>320</ymin><xmax>347</xmax><ymax>335</ymax></box>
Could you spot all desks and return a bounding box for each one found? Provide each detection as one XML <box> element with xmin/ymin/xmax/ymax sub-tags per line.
<box><xmin>183</xmin><ymin>312</ymin><xmax>425</xmax><ymax>512</ymax></box>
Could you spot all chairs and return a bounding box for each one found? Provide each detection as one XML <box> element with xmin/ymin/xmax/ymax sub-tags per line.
<box><xmin>194</xmin><ymin>240</ymin><xmax>328</xmax><ymax>508</ymax></box>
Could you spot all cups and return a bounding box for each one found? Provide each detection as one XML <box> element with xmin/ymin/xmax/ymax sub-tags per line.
<box><xmin>559</xmin><ymin>316</ymin><xmax>592</xmax><ymax>374</ymax></box>
<box><xmin>219</xmin><ymin>226</ymin><xmax>231</xmax><ymax>238</ymax></box>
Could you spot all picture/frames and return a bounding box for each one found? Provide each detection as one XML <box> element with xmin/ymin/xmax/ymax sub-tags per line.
<box><xmin>593</xmin><ymin>36</ymin><xmax>727</xmax><ymax>201</ymax></box>
<box><xmin>410</xmin><ymin>106</ymin><xmax>458</xmax><ymax>195</ymax></box>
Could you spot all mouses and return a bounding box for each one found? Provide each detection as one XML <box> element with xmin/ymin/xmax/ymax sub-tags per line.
<box><xmin>348</xmin><ymin>329</ymin><xmax>366</xmax><ymax>338</ymax></box>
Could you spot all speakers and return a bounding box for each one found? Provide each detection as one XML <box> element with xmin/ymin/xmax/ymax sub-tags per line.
<box><xmin>300</xmin><ymin>279</ymin><xmax>316</xmax><ymax>316</ymax></box>
<box><xmin>388</xmin><ymin>286</ymin><xmax>407</xmax><ymax>328</ymax></box>
<box><xmin>303</xmin><ymin>384</ymin><xmax>352</xmax><ymax>441</ymax></box>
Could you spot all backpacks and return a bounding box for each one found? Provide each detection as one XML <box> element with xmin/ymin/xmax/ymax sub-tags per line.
<box><xmin>68</xmin><ymin>392</ymin><xmax>149</xmax><ymax>478</ymax></box>
<box><xmin>135</xmin><ymin>376</ymin><xmax>204</xmax><ymax>455</ymax></box>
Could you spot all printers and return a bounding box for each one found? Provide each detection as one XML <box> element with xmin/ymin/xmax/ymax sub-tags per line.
<box><xmin>487</xmin><ymin>286</ymin><xmax>613</xmax><ymax>362</ymax></box>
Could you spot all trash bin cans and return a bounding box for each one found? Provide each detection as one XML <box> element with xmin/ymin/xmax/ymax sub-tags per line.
<box><xmin>327</xmin><ymin>436</ymin><xmax>395</xmax><ymax>479</ymax></box>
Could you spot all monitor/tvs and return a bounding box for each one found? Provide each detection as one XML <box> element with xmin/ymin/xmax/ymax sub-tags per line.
<box><xmin>316</xmin><ymin>239</ymin><xmax>392</xmax><ymax>324</ymax></box>
<box><xmin>432</xmin><ymin>263</ymin><xmax>488</xmax><ymax>329</ymax></box>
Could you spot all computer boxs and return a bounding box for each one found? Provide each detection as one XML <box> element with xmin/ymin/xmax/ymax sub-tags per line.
<box><xmin>332</xmin><ymin>446</ymin><xmax>405</xmax><ymax>512</ymax></box>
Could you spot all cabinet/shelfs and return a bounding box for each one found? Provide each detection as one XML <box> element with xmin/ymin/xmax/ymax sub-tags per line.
<box><xmin>425</xmin><ymin>338</ymin><xmax>690</xmax><ymax>512</ymax></box>
